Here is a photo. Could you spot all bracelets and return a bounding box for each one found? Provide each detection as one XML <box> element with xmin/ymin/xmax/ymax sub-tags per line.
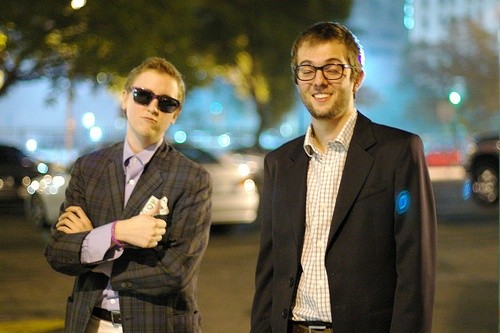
<box><xmin>111</xmin><ymin>220</ymin><xmax>127</xmax><ymax>248</ymax></box>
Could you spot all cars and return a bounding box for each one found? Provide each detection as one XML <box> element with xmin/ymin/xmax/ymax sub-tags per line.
<box><xmin>0</xmin><ymin>134</ymin><xmax>500</xmax><ymax>238</ymax></box>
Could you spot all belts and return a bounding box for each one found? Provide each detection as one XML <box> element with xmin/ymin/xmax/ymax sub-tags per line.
<box><xmin>91</xmin><ymin>307</ymin><xmax>124</xmax><ymax>327</ymax></box>
<box><xmin>299</xmin><ymin>318</ymin><xmax>331</xmax><ymax>333</ymax></box>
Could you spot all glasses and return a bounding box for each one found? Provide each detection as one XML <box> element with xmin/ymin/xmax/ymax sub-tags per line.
<box><xmin>128</xmin><ymin>86</ymin><xmax>182</xmax><ymax>114</ymax></box>
<box><xmin>292</xmin><ymin>64</ymin><xmax>352</xmax><ymax>80</ymax></box>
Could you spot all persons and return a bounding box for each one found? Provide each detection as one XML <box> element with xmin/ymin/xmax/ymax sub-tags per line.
<box><xmin>43</xmin><ymin>56</ymin><xmax>213</xmax><ymax>333</ymax></box>
<box><xmin>249</xmin><ymin>21</ymin><xmax>438</xmax><ymax>333</ymax></box>
